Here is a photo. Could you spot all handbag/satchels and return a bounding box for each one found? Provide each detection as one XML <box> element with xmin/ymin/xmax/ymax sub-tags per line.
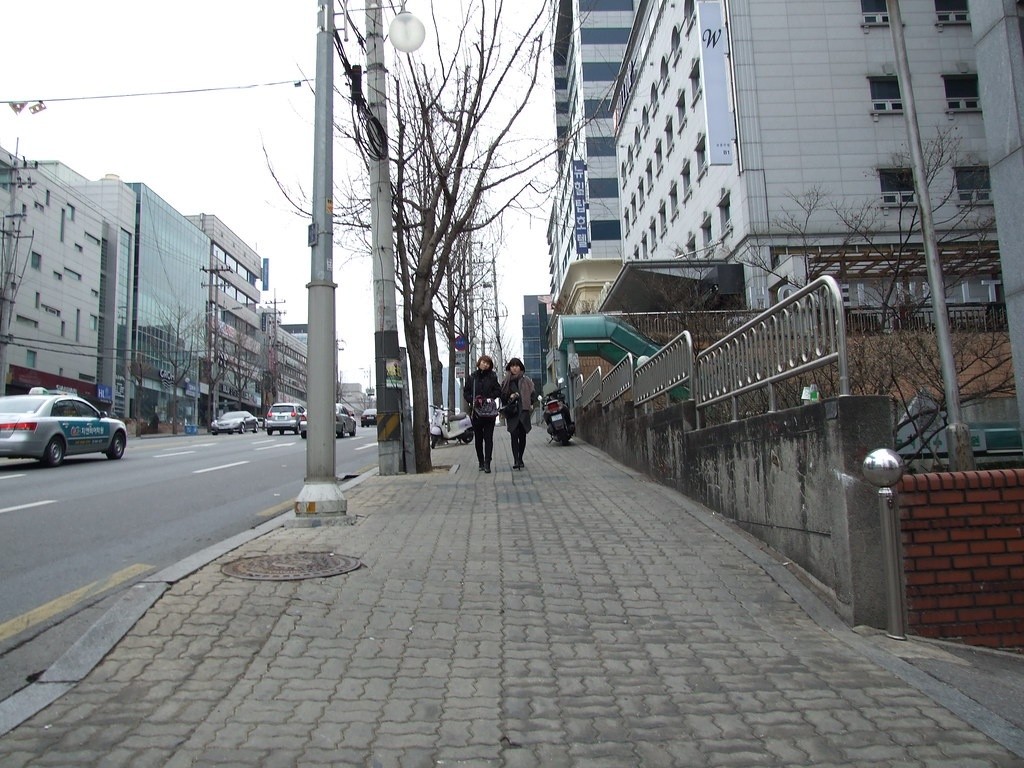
<box><xmin>497</xmin><ymin>396</ymin><xmax>521</xmax><ymax>419</ymax></box>
<box><xmin>474</xmin><ymin>395</ymin><xmax>499</xmax><ymax>418</ymax></box>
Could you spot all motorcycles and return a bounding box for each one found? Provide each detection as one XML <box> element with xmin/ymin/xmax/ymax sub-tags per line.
<box><xmin>536</xmin><ymin>377</ymin><xmax>575</xmax><ymax>447</ymax></box>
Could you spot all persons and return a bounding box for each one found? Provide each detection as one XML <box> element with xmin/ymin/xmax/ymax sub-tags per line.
<box><xmin>500</xmin><ymin>359</ymin><xmax>536</xmax><ymax>471</ymax></box>
<box><xmin>463</xmin><ymin>356</ymin><xmax>502</xmax><ymax>473</ymax></box>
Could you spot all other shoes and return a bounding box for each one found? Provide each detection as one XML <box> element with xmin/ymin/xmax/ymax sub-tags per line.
<box><xmin>518</xmin><ymin>449</ymin><xmax>524</xmax><ymax>467</ymax></box>
<box><xmin>484</xmin><ymin>458</ymin><xmax>492</xmax><ymax>473</ymax></box>
<box><xmin>513</xmin><ymin>453</ymin><xmax>520</xmax><ymax>469</ymax></box>
<box><xmin>479</xmin><ymin>456</ymin><xmax>485</xmax><ymax>471</ymax></box>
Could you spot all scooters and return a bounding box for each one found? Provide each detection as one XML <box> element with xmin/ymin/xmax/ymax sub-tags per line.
<box><xmin>429</xmin><ymin>404</ymin><xmax>474</xmax><ymax>450</ymax></box>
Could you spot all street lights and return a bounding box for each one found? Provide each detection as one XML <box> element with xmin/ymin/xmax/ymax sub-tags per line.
<box><xmin>339</xmin><ymin>367</ymin><xmax>364</xmax><ymax>402</ymax></box>
<box><xmin>284</xmin><ymin>0</ymin><xmax>427</xmax><ymax>530</ymax></box>
<box><xmin>194</xmin><ymin>306</ymin><xmax>244</xmax><ymax>426</ymax></box>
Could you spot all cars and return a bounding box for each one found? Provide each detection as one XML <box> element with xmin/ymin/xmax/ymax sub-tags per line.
<box><xmin>299</xmin><ymin>402</ymin><xmax>357</xmax><ymax>439</ymax></box>
<box><xmin>265</xmin><ymin>401</ymin><xmax>307</xmax><ymax>435</ymax></box>
<box><xmin>257</xmin><ymin>416</ymin><xmax>267</xmax><ymax>428</ymax></box>
<box><xmin>211</xmin><ymin>410</ymin><xmax>260</xmax><ymax>435</ymax></box>
<box><xmin>360</xmin><ymin>408</ymin><xmax>377</xmax><ymax>427</ymax></box>
<box><xmin>0</xmin><ymin>386</ymin><xmax>128</xmax><ymax>467</ymax></box>
